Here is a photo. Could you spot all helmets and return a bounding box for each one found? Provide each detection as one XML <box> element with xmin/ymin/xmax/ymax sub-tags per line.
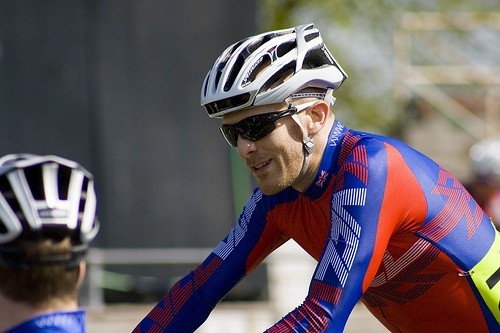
<box><xmin>0</xmin><ymin>151</ymin><xmax>101</xmax><ymax>269</ymax></box>
<box><xmin>200</xmin><ymin>23</ymin><xmax>349</xmax><ymax>118</ymax></box>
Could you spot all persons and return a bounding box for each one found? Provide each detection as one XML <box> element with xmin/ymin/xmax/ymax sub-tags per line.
<box><xmin>0</xmin><ymin>154</ymin><xmax>100</xmax><ymax>333</ymax></box>
<box><xmin>132</xmin><ymin>22</ymin><xmax>500</xmax><ymax>333</ymax></box>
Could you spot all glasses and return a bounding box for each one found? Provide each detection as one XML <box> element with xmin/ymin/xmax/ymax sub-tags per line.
<box><xmin>219</xmin><ymin>99</ymin><xmax>321</xmax><ymax>148</ymax></box>
